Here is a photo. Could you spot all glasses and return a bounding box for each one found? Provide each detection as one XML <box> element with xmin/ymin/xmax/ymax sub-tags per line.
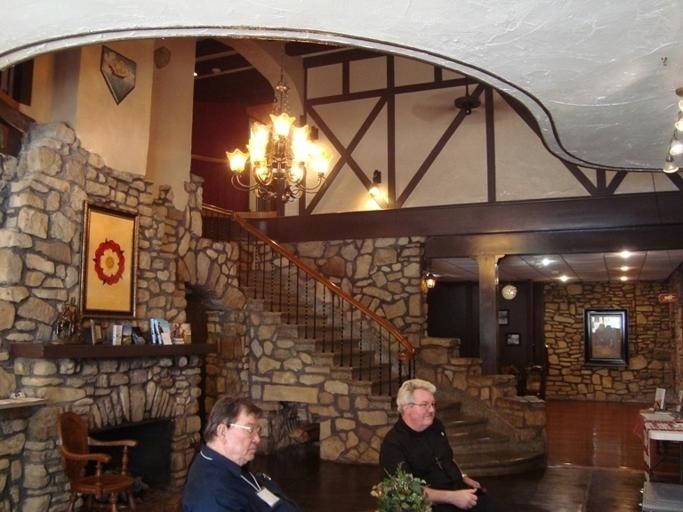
<box><xmin>229</xmin><ymin>423</ymin><xmax>262</xmax><ymax>434</ymax></box>
<box><xmin>413</xmin><ymin>402</ymin><xmax>435</xmax><ymax>408</ymax></box>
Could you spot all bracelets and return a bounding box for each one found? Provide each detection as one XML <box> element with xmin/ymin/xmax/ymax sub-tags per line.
<box><xmin>460</xmin><ymin>472</ymin><xmax>469</xmax><ymax>479</ymax></box>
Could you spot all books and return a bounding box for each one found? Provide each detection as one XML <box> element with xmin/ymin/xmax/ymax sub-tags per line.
<box><xmin>653</xmin><ymin>386</ymin><xmax>666</xmax><ymax>410</ymax></box>
<box><xmin>110</xmin><ymin>316</ymin><xmax>191</xmax><ymax>346</ymax></box>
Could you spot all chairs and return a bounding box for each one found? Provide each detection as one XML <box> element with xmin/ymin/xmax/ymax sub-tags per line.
<box><xmin>55</xmin><ymin>411</ymin><xmax>138</xmax><ymax>511</ymax></box>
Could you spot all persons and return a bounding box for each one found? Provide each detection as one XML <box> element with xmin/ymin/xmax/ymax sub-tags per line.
<box><xmin>61</xmin><ymin>295</ymin><xmax>81</xmax><ymax>340</ymax></box>
<box><xmin>374</xmin><ymin>379</ymin><xmax>489</xmax><ymax>512</ymax></box>
<box><xmin>176</xmin><ymin>394</ymin><xmax>297</xmax><ymax>511</ymax></box>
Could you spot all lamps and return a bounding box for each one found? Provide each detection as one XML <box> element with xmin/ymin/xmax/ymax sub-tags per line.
<box><xmin>424</xmin><ymin>268</ymin><xmax>436</xmax><ymax>289</ymax></box>
<box><xmin>453</xmin><ymin>76</ymin><xmax>481</xmax><ymax>115</ymax></box>
<box><xmin>225</xmin><ymin>45</ymin><xmax>332</xmax><ymax>204</ymax></box>
<box><xmin>663</xmin><ymin>97</ymin><xmax>683</xmax><ymax>174</ymax></box>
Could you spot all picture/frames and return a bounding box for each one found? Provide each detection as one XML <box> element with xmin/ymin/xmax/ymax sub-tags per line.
<box><xmin>498</xmin><ymin>309</ymin><xmax>510</xmax><ymax>326</ymax></box>
<box><xmin>583</xmin><ymin>309</ymin><xmax>627</xmax><ymax>368</ymax></box>
<box><xmin>504</xmin><ymin>333</ymin><xmax>520</xmax><ymax>347</ymax></box>
<box><xmin>89</xmin><ymin>319</ymin><xmax>103</xmax><ymax>346</ymax></box>
<box><xmin>80</xmin><ymin>202</ymin><xmax>143</xmax><ymax>321</ymax></box>
